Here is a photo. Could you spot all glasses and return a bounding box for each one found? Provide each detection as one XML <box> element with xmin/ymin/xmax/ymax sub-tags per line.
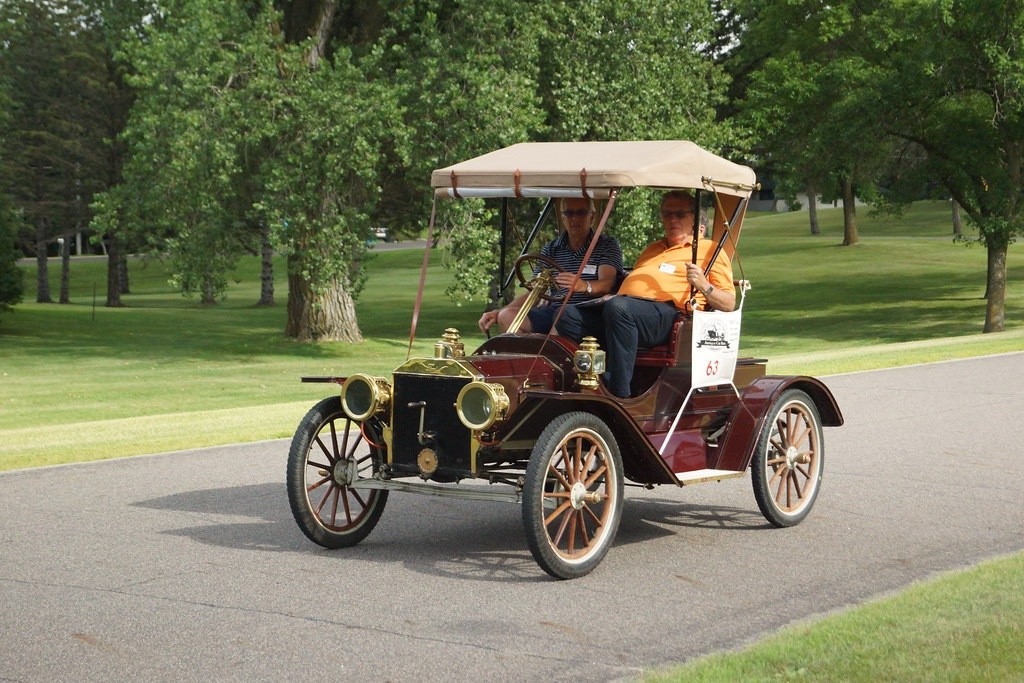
<box><xmin>560</xmin><ymin>208</ymin><xmax>593</xmax><ymax>218</ymax></box>
<box><xmin>663</xmin><ymin>210</ymin><xmax>696</xmax><ymax>219</ymax></box>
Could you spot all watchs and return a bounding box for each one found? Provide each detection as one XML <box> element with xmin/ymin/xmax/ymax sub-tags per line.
<box><xmin>586</xmin><ymin>280</ymin><xmax>593</xmax><ymax>294</ymax></box>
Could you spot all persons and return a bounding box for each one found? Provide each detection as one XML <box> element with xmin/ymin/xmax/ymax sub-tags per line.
<box><xmin>552</xmin><ymin>189</ymin><xmax>736</xmax><ymax>395</ymax></box>
<box><xmin>477</xmin><ymin>199</ymin><xmax>623</xmax><ymax>347</ymax></box>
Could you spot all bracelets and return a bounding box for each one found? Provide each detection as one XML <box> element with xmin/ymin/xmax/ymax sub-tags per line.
<box><xmin>703</xmin><ymin>284</ymin><xmax>713</xmax><ymax>296</ymax></box>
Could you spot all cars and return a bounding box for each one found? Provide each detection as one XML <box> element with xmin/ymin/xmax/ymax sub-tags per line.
<box><xmin>287</xmin><ymin>142</ymin><xmax>844</xmax><ymax>578</ymax></box>
<box><xmin>371</xmin><ymin>228</ymin><xmax>391</xmax><ymax>242</ymax></box>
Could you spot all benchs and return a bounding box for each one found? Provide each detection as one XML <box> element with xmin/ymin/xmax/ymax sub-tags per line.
<box><xmin>598</xmin><ymin>273</ymin><xmax>727</xmax><ymax>367</ymax></box>
